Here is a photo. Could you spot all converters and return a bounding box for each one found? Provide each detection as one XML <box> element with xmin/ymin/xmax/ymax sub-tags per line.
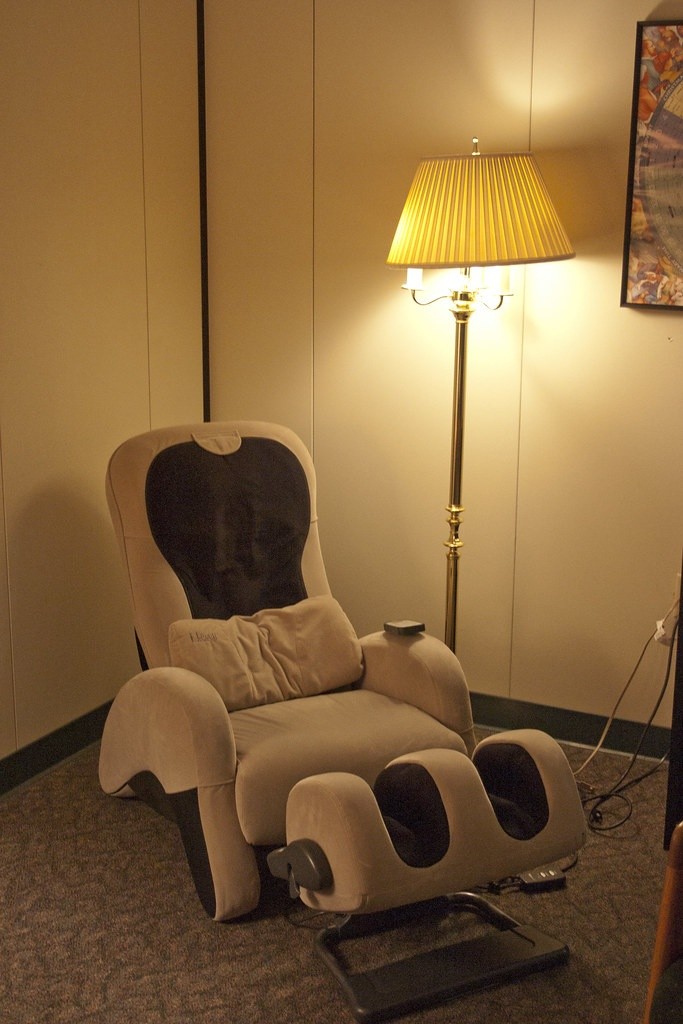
<box><xmin>519</xmin><ymin>867</ymin><xmax>566</xmax><ymax>893</ymax></box>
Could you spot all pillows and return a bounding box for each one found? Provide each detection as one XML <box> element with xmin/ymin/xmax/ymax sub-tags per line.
<box><xmin>166</xmin><ymin>595</ymin><xmax>366</xmax><ymax>714</ymax></box>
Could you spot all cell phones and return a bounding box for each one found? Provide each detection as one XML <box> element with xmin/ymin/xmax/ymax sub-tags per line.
<box><xmin>382</xmin><ymin>620</ymin><xmax>424</xmax><ymax>636</ymax></box>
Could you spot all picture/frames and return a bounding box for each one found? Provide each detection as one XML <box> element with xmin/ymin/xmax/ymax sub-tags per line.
<box><xmin>622</xmin><ymin>18</ymin><xmax>681</xmax><ymax>314</ymax></box>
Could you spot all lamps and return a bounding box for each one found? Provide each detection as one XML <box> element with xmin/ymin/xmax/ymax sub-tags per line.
<box><xmin>387</xmin><ymin>134</ymin><xmax>578</xmax><ymax>651</ymax></box>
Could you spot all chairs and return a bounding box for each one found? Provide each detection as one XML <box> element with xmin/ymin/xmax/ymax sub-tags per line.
<box><xmin>98</xmin><ymin>423</ymin><xmax>585</xmax><ymax>924</ymax></box>
